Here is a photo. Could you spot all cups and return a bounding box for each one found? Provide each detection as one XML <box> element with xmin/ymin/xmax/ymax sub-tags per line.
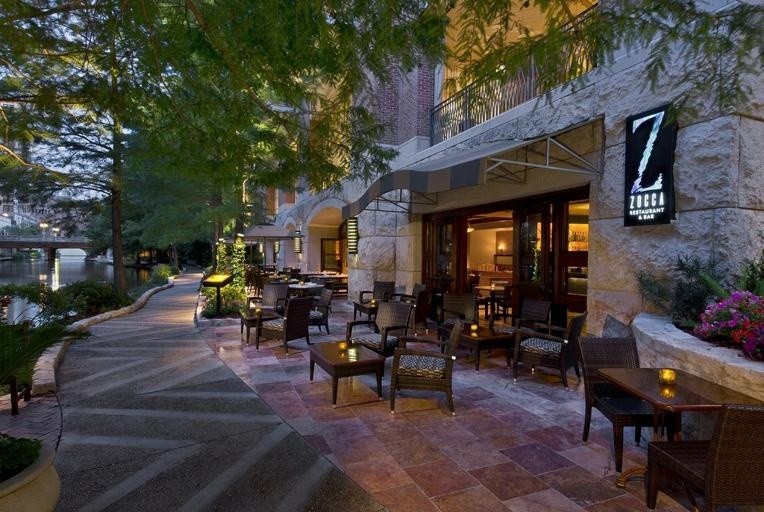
<box><xmin>472</xmin><ymin>262</ymin><xmax>495</xmax><ymax>271</ymax></box>
<box><xmin>307</xmin><ymin>269</ymin><xmax>339</xmax><ymax>277</ymax></box>
<box><xmin>470</xmin><ymin>332</ymin><xmax>478</xmax><ymax>337</ymax></box>
<box><xmin>656</xmin><ymin>387</ymin><xmax>678</xmax><ymax>401</ymax></box>
<box><xmin>346</xmin><ymin>343</ymin><xmax>359</xmax><ymax>359</ymax></box>
<box><xmin>470</xmin><ymin>323</ymin><xmax>478</xmax><ymax>331</ymax></box>
<box><xmin>337</xmin><ymin>341</ymin><xmax>347</xmax><ymax>352</ymax></box>
<box><xmin>255</xmin><ymin>307</ymin><xmax>261</xmax><ymax>314</ymax></box>
<box><xmin>264</xmin><ymin>269</ymin><xmax>304</xmax><ymax>286</ymax></box>
<box><xmin>336</xmin><ymin>351</ymin><xmax>348</xmax><ymax>361</ymax></box>
<box><xmin>368</xmin><ymin>296</ymin><xmax>376</xmax><ymax>304</ymax></box>
<box><xmin>657</xmin><ymin>368</ymin><xmax>676</xmax><ymax>385</ymax></box>
<box><xmin>490</xmin><ymin>280</ymin><xmax>495</xmax><ymax>287</ymax></box>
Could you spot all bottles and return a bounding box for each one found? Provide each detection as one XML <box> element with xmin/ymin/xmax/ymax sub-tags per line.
<box><xmin>569</xmin><ymin>231</ymin><xmax>585</xmax><ymax>241</ymax></box>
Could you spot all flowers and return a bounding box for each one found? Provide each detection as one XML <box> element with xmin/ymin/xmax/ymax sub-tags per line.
<box><xmin>694</xmin><ymin>290</ymin><xmax>764</xmax><ymax>362</ymax></box>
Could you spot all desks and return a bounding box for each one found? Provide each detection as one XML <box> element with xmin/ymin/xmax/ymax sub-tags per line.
<box><xmin>309</xmin><ymin>341</ymin><xmax>386</xmax><ymax>409</ymax></box>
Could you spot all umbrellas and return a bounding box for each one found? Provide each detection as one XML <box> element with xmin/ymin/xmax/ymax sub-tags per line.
<box><xmin>219</xmin><ymin>232</ymin><xmax>264</xmax><ymax>269</ymax></box>
<box><xmin>229</xmin><ymin>220</ymin><xmax>304</xmax><ymax>273</ymax></box>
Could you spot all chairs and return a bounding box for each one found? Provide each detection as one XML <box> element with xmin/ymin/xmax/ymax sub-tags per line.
<box><xmin>390</xmin><ymin>319</ymin><xmax>464</xmax><ymax>415</ymax></box>
<box><xmin>577</xmin><ymin>335</ymin><xmax>665</xmax><ymax>472</ymax></box>
<box><xmin>644</xmin><ymin>402</ymin><xmax>764</xmax><ymax>511</ymax></box>
<box><xmin>436</xmin><ymin>290</ymin><xmax>588</xmax><ymax>391</ymax></box>
<box><xmin>346</xmin><ymin>302</ymin><xmax>415</xmax><ymax>379</ymax></box>
<box><xmin>352</xmin><ymin>281</ymin><xmax>430</xmax><ymax>336</ymax></box>
<box><xmin>239</xmin><ymin>264</ymin><xmax>334</xmax><ymax>357</ymax></box>
